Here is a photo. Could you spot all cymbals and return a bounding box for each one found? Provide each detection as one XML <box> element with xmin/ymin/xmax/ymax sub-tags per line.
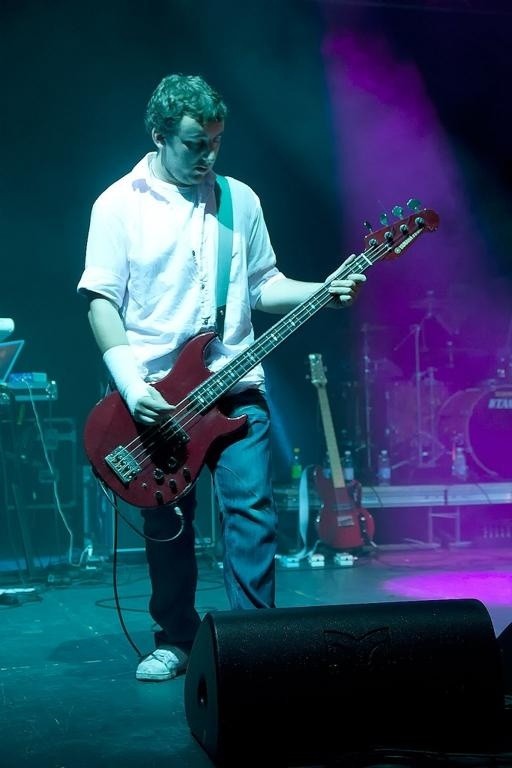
<box><xmin>407</xmin><ymin>298</ymin><xmax>455</xmax><ymax>311</ymax></box>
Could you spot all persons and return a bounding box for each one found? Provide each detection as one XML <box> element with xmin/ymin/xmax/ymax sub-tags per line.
<box><xmin>75</xmin><ymin>74</ymin><xmax>368</xmax><ymax>682</ymax></box>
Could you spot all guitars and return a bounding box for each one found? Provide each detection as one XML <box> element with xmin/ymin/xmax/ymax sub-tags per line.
<box><xmin>83</xmin><ymin>197</ymin><xmax>440</xmax><ymax>505</ymax></box>
<box><xmin>305</xmin><ymin>353</ymin><xmax>375</xmax><ymax>550</ymax></box>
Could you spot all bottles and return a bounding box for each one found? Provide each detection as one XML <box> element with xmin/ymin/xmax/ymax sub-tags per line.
<box><xmin>377</xmin><ymin>451</ymin><xmax>392</xmax><ymax>488</ymax></box>
<box><xmin>320</xmin><ymin>450</ymin><xmax>356</xmax><ymax>488</ymax></box>
<box><xmin>288</xmin><ymin>447</ymin><xmax>303</xmax><ymax>492</ymax></box>
<box><xmin>494</xmin><ymin>357</ymin><xmax>506</xmax><ymax>385</ymax></box>
<box><xmin>448</xmin><ymin>433</ymin><xmax>470</xmax><ymax>485</ymax></box>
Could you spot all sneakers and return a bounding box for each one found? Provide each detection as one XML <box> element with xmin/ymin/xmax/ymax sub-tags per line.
<box><xmin>135</xmin><ymin>640</ymin><xmax>190</xmax><ymax>681</ymax></box>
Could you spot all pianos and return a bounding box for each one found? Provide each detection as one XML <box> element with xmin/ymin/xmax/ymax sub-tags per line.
<box><xmin>0</xmin><ymin>379</ymin><xmax>58</xmax><ymax>404</ymax></box>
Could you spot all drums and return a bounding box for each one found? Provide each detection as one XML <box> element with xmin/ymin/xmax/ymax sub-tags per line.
<box><xmin>436</xmin><ymin>383</ymin><xmax>512</xmax><ymax>482</ymax></box>
<box><xmin>384</xmin><ymin>374</ymin><xmax>449</xmax><ymax>457</ymax></box>
